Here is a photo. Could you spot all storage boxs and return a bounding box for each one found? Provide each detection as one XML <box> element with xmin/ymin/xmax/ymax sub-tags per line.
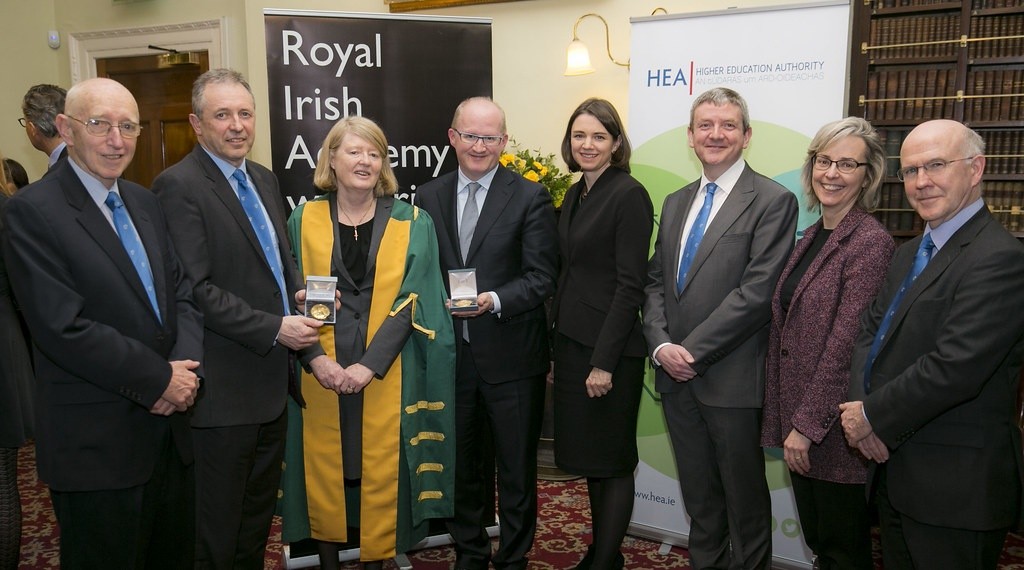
<box><xmin>304</xmin><ymin>275</ymin><xmax>338</xmax><ymax>325</ymax></box>
<box><xmin>446</xmin><ymin>268</ymin><xmax>479</xmax><ymax>312</ymax></box>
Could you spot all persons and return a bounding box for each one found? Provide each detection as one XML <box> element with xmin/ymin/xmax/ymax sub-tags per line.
<box><xmin>0</xmin><ymin>160</ymin><xmax>34</xmax><ymax>568</ymax></box>
<box><xmin>839</xmin><ymin>119</ymin><xmax>1024</xmax><ymax>570</ymax></box>
<box><xmin>281</xmin><ymin>118</ymin><xmax>455</xmax><ymax>570</ymax></box>
<box><xmin>415</xmin><ymin>97</ymin><xmax>557</xmax><ymax>570</ymax></box>
<box><xmin>639</xmin><ymin>88</ymin><xmax>799</xmax><ymax>570</ymax></box>
<box><xmin>19</xmin><ymin>83</ymin><xmax>68</xmax><ymax>170</ymax></box>
<box><xmin>545</xmin><ymin>98</ymin><xmax>654</xmax><ymax>570</ymax></box>
<box><xmin>151</xmin><ymin>70</ymin><xmax>323</xmax><ymax>570</ymax></box>
<box><xmin>762</xmin><ymin>117</ymin><xmax>897</xmax><ymax>570</ymax></box>
<box><xmin>0</xmin><ymin>76</ymin><xmax>203</xmax><ymax>570</ymax></box>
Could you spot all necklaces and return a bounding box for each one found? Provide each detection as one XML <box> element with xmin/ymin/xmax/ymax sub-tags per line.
<box><xmin>338</xmin><ymin>200</ymin><xmax>373</xmax><ymax>241</ymax></box>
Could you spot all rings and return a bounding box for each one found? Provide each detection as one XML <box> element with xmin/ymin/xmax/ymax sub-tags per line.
<box><xmin>796</xmin><ymin>457</ymin><xmax>800</xmax><ymax>459</ymax></box>
<box><xmin>349</xmin><ymin>385</ymin><xmax>355</xmax><ymax>389</ymax></box>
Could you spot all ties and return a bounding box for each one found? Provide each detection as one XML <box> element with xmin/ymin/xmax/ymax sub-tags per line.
<box><xmin>459</xmin><ymin>183</ymin><xmax>481</xmax><ymax>342</ymax></box>
<box><xmin>106</xmin><ymin>191</ymin><xmax>163</xmax><ymax>327</ymax></box>
<box><xmin>677</xmin><ymin>182</ymin><xmax>717</xmax><ymax>295</ymax></box>
<box><xmin>232</xmin><ymin>169</ymin><xmax>291</xmax><ymax>317</ymax></box>
<box><xmin>865</xmin><ymin>233</ymin><xmax>936</xmax><ymax>392</ymax></box>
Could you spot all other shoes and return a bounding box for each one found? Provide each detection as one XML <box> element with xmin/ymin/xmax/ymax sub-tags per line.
<box><xmin>573</xmin><ymin>545</ymin><xmax>624</xmax><ymax>570</ymax></box>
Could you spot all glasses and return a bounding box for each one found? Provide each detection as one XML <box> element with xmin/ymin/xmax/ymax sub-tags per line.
<box><xmin>897</xmin><ymin>156</ymin><xmax>973</xmax><ymax>182</ymax></box>
<box><xmin>18</xmin><ymin>118</ymin><xmax>29</xmax><ymax>127</ymax></box>
<box><xmin>812</xmin><ymin>155</ymin><xmax>871</xmax><ymax>174</ymax></box>
<box><xmin>454</xmin><ymin>128</ymin><xmax>504</xmax><ymax>146</ymax></box>
<box><xmin>69</xmin><ymin>116</ymin><xmax>142</xmax><ymax>139</ymax></box>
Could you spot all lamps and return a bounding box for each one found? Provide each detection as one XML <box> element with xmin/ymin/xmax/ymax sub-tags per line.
<box><xmin>561</xmin><ymin>9</ymin><xmax>632</xmax><ymax>81</ymax></box>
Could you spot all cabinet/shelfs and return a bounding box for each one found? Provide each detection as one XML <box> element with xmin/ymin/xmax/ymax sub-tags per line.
<box><xmin>846</xmin><ymin>0</ymin><xmax>1024</xmax><ymax>246</ymax></box>
